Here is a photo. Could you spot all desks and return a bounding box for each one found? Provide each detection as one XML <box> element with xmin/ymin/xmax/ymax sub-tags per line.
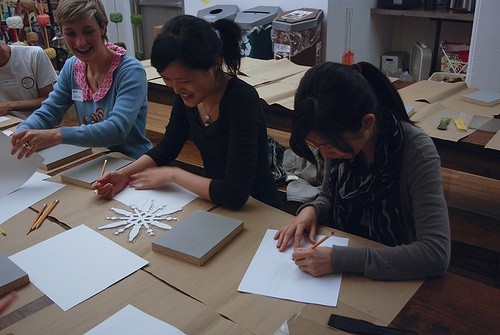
<box><xmin>0</xmin><ymin>57</ymin><xmax>500</xmax><ymax>335</ymax></box>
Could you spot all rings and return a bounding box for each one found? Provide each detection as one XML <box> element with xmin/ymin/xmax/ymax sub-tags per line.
<box><xmin>25</xmin><ymin>142</ymin><xmax>31</xmax><ymax>150</ymax></box>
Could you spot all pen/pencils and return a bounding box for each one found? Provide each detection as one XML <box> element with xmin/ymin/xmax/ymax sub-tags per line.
<box><xmin>312</xmin><ymin>230</ymin><xmax>335</xmax><ymax>249</ymax></box>
<box><xmin>32</xmin><ymin>201</ymin><xmax>55</xmax><ymax>231</ymax></box>
<box><xmin>96</xmin><ymin>159</ymin><xmax>108</xmax><ymax>196</ymax></box>
<box><xmin>0</xmin><ymin>226</ymin><xmax>7</xmax><ymax>236</ymax></box>
<box><xmin>26</xmin><ymin>203</ymin><xmax>47</xmax><ymax>235</ymax></box>
<box><xmin>36</xmin><ymin>199</ymin><xmax>59</xmax><ymax>229</ymax></box>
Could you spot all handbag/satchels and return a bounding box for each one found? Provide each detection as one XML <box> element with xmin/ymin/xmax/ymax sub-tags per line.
<box><xmin>267</xmin><ymin>133</ymin><xmax>286</xmax><ymax>185</ymax></box>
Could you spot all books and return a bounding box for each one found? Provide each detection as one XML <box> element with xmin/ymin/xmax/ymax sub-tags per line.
<box><xmin>29</xmin><ymin>144</ymin><xmax>92</xmax><ymax>171</ymax></box>
<box><xmin>402</xmin><ymin>104</ymin><xmax>415</xmax><ymax>117</ymax></box>
<box><xmin>151</xmin><ymin>210</ymin><xmax>245</xmax><ymax>265</ymax></box>
<box><xmin>60</xmin><ymin>154</ymin><xmax>132</xmax><ymax>188</ymax></box>
<box><xmin>461</xmin><ymin>88</ymin><xmax>500</xmax><ymax>106</ymax></box>
<box><xmin>0</xmin><ymin>252</ymin><xmax>30</xmax><ymax>298</ymax></box>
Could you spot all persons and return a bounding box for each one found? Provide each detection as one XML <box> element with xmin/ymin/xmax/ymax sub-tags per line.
<box><xmin>12</xmin><ymin>0</ymin><xmax>155</xmax><ymax>159</ymax></box>
<box><xmin>0</xmin><ymin>290</ymin><xmax>17</xmax><ymax>335</ymax></box>
<box><xmin>0</xmin><ymin>41</ymin><xmax>58</xmax><ymax>126</ymax></box>
<box><xmin>273</xmin><ymin>59</ymin><xmax>452</xmax><ymax>280</ymax></box>
<box><xmin>90</xmin><ymin>13</ymin><xmax>277</xmax><ymax>210</ymax></box>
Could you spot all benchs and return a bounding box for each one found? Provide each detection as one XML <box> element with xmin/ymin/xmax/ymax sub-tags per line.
<box><xmin>63</xmin><ymin>82</ymin><xmax>500</xmax><ymax>280</ymax></box>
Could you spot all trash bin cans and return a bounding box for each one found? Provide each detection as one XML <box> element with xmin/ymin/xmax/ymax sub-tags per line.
<box><xmin>234</xmin><ymin>6</ymin><xmax>283</xmax><ymax>60</ymax></box>
<box><xmin>272</xmin><ymin>7</ymin><xmax>324</xmax><ymax>68</ymax></box>
<box><xmin>196</xmin><ymin>5</ymin><xmax>240</xmax><ymax>39</ymax></box>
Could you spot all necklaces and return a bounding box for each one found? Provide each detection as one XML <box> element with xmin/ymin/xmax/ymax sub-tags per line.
<box><xmin>201</xmin><ymin>101</ymin><xmax>220</xmax><ymax>126</ymax></box>
<box><xmin>87</xmin><ymin>58</ymin><xmax>108</xmax><ymax>89</ymax></box>
